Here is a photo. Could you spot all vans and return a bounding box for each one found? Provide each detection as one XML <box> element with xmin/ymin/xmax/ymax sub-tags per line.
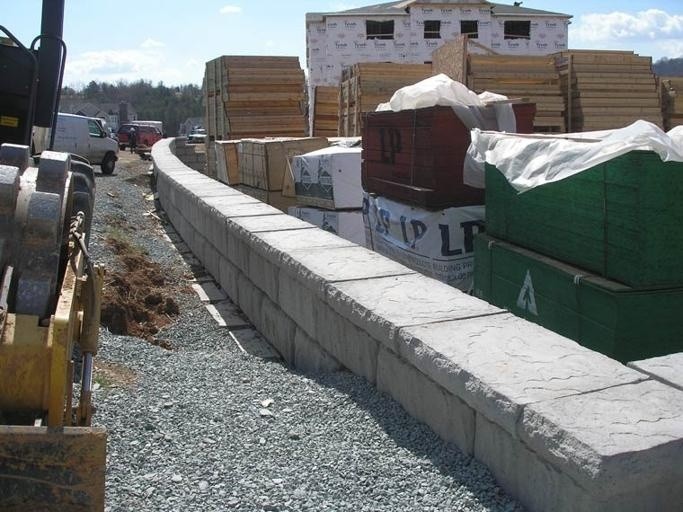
<box><xmin>108</xmin><ymin>121</ymin><xmax>163</xmax><ymax>151</ymax></box>
<box><xmin>46</xmin><ymin>113</ymin><xmax>119</xmax><ymax>174</ymax></box>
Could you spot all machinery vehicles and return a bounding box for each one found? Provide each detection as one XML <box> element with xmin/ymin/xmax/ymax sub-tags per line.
<box><xmin>0</xmin><ymin>0</ymin><xmax>107</xmax><ymax>512</ymax></box>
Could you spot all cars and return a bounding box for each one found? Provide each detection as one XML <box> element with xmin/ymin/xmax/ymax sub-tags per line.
<box><xmin>188</xmin><ymin>129</ymin><xmax>207</xmax><ymax>144</ymax></box>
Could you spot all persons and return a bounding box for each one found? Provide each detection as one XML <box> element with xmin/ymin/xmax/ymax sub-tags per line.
<box><xmin>128</xmin><ymin>127</ymin><xmax>135</xmax><ymax>153</ymax></box>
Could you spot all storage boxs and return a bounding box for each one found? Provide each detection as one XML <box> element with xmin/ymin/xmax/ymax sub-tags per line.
<box><xmin>239</xmin><ymin>135</ymin><xmax>327</xmax><ymax>213</ymax></box>
<box><xmin>287</xmin><ymin>145</ymin><xmax>362</xmax><ymax>245</ymax></box>
<box><xmin>215</xmin><ymin>136</ymin><xmax>240</xmax><ymax>187</ymax></box>
<box><xmin>472</xmin><ymin>119</ymin><xmax>683</xmax><ymax>365</ymax></box>
<box><xmin>361</xmin><ymin>101</ymin><xmax>538</xmax><ymax>300</ymax></box>
